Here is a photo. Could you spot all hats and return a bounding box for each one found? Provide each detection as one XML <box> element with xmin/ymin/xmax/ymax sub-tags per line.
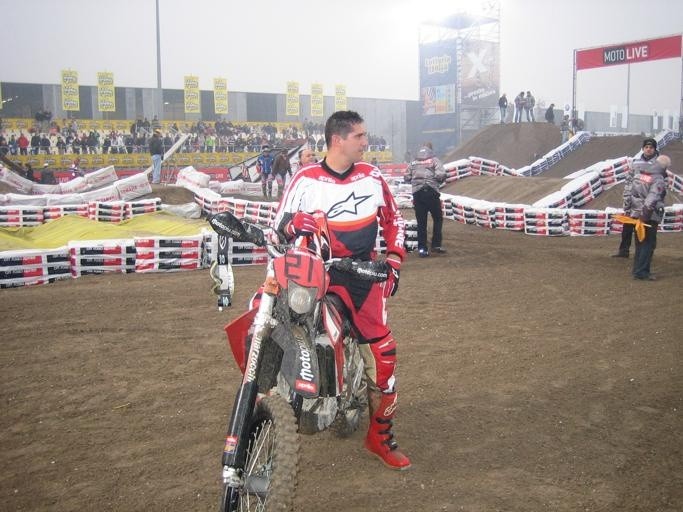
<box><xmin>281</xmin><ymin>148</ymin><xmax>288</xmax><ymax>154</ymax></box>
<box><xmin>643</xmin><ymin>138</ymin><xmax>658</xmax><ymax>148</ymax></box>
<box><xmin>262</xmin><ymin>145</ymin><xmax>270</xmax><ymax>152</ymax></box>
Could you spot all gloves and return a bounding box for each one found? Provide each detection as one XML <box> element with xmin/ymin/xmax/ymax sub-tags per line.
<box><xmin>380</xmin><ymin>258</ymin><xmax>401</xmax><ymax>297</ymax></box>
<box><xmin>287</xmin><ymin>210</ymin><xmax>318</xmax><ymax>235</ymax></box>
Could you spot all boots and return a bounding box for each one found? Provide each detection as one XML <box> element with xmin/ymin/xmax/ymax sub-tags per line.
<box><xmin>365</xmin><ymin>385</ymin><xmax>410</xmax><ymax>469</ymax></box>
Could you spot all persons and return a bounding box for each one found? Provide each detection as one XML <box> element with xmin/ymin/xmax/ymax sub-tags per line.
<box><xmin>1</xmin><ymin>114</ymin><xmax>387</xmax><ymax>156</ymax></box>
<box><xmin>257</xmin><ymin>146</ymin><xmax>293</xmax><ymax>201</ymax></box>
<box><xmin>609</xmin><ymin>137</ymin><xmax>660</xmax><ymax>257</ymax></box>
<box><xmin>1</xmin><ymin>166</ymin><xmax>12</xmax><ymax>178</ymax></box>
<box><xmin>403</xmin><ymin>141</ymin><xmax>447</xmax><ymax>258</ymax></box>
<box><xmin>292</xmin><ymin>149</ymin><xmax>317</xmax><ymax>174</ymax></box>
<box><xmin>545</xmin><ymin>103</ymin><xmax>554</xmax><ymax>123</ymax></box>
<box><xmin>498</xmin><ymin>92</ymin><xmax>507</xmax><ymax>123</ymax></box>
<box><xmin>17</xmin><ymin>161</ymin><xmax>56</xmax><ymax>185</ymax></box>
<box><xmin>371</xmin><ymin>157</ymin><xmax>381</xmax><ymax>171</ymax></box>
<box><xmin>628</xmin><ymin>155</ymin><xmax>671</xmax><ymax>281</ymax></box>
<box><xmin>514</xmin><ymin>91</ymin><xmax>524</xmax><ymax>123</ymax></box>
<box><xmin>249</xmin><ymin>109</ymin><xmax>413</xmax><ymax>473</ymax></box>
<box><xmin>559</xmin><ymin>114</ymin><xmax>571</xmax><ymax>144</ymax></box>
<box><xmin>72</xmin><ymin>158</ymin><xmax>81</xmax><ymax>177</ymax></box>
<box><xmin>523</xmin><ymin>91</ymin><xmax>535</xmax><ymax>122</ymax></box>
<box><xmin>149</xmin><ymin>132</ymin><xmax>164</xmax><ymax>183</ymax></box>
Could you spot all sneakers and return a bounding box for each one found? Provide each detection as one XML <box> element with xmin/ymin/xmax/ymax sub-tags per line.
<box><xmin>432</xmin><ymin>246</ymin><xmax>446</xmax><ymax>252</ymax></box>
<box><xmin>634</xmin><ymin>271</ymin><xmax>656</xmax><ymax>282</ymax></box>
<box><xmin>418</xmin><ymin>249</ymin><xmax>428</xmax><ymax>257</ymax></box>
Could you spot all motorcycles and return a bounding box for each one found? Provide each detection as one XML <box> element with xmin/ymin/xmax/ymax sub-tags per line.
<box><xmin>208</xmin><ymin>207</ymin><xmax>390</xmax><ymax>511</ymax></box>
